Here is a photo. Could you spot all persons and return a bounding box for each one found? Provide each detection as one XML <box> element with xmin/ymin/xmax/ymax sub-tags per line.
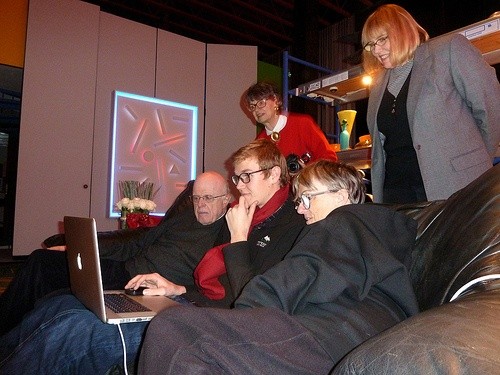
<box><xmin>137</xmin><ymin>160</ymin><xmax>420</xmax><ymax>374</ymax></box>
<box><xmin>0</xmin><ymin>138</ymin><xmax>306</xmax><ymax>375</ymax></box>
<box><xmin>246</xmin><ymin>79</ymin><xmax>338</xmax><ymax>172</ymax></box>
<box><xmin>0</xmin><ymin>170</ymin><xmax>232</xmax><ymax>351</ymax></box>
<box><xmin>361</xmin><ymin>3</ymin><xmax>500</xmax><ymax>202</ymax></box>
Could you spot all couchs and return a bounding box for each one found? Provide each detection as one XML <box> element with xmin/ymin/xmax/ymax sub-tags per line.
<box><xmin>44</xmin><ymin>165</ymin><xmax>499</xmax><ymax>374</ymax></box>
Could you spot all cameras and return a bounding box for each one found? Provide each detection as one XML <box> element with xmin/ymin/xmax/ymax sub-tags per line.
<box><xmin>286</xmin><ymin>153</ymin><xmax>310</xmax><ymax>173</ymax></box>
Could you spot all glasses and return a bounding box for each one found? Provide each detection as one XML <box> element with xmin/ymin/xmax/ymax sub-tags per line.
<box><xmin>231</xmin><ymin>168</ymin><xmax>270</xmax><ymax>186</ymax></box>
<box><xmin>245</xmin><ymin>98</ymin><xmax>267</xmax><ymax>113</ymax></box>
<box><xmin>295</xmin><ymin>187</ymin><xmax>338</xmax><ymax>210</ymax></box>
<box><xmin>188</xmin><ymin>194</ymin><xmax>225</xmax><ymax>203</ymax></box>
<box><xmin>363</xmin><ymin>35</ymin><xmax>389</xmax><ymax>52</ymax></box>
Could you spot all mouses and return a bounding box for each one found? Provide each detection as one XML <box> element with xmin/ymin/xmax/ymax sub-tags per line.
<box><xmin>124</xmin><ymin>286</ymin><xmax>148</xmax><ymax>296</ymax></box>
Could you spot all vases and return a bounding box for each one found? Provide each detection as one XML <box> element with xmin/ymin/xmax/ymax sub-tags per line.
<box><xmin>122</xmin><ymin>209</ymin><xmax>147</xmax><ymax>230</ymax></box>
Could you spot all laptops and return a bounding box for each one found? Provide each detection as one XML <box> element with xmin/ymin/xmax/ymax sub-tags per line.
<box><xmin>63</xmin><ymin>215</ymin><xmax>181</xmax><ymax>325</ymax></box>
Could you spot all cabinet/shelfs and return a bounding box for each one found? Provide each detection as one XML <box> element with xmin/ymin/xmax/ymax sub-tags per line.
<box><xmin>283</xmin><ymin>15</ymin><xmax>500</xmax><ymax>165</ymax></box>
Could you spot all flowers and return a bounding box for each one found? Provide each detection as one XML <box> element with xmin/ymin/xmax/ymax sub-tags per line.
<box><xmin>116</xmin><ymin>198</ymin><xmax>156</xmax><ymax>212</ymax></box>
